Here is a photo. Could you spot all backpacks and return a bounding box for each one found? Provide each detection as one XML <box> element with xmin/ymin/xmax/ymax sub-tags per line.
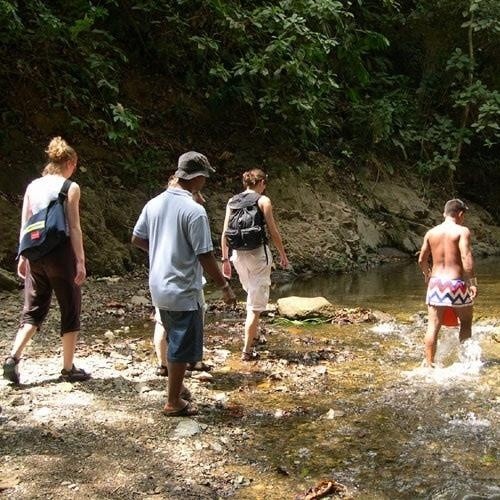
<box><xmin>224</xmin><ymin>193</ymin><xmax>268</xmax><ymax>249</ymax></box>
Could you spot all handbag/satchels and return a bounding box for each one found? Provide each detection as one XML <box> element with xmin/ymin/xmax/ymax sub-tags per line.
<box><xmin>16</xmin><ymin>200</ymin><xmax>70</xmax><ymax>263</ymax></box>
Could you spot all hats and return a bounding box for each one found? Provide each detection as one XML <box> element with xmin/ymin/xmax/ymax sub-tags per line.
<box><xmin>175</xmin><ymin>151</ymin><xmax>216</xmax><ymax>180</ymax></box>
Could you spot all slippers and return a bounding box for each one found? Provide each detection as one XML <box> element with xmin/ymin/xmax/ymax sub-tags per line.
<box><xmin>156</xmin><ymin>361</ymin><xmax>211</xmax><ymax>417</ymax></box>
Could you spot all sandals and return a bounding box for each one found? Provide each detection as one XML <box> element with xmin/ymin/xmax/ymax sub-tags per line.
<box><xmin>60</xmin><ymin>364</ymin><xmax>86</xmax><ymax>378</ymax></box>
<box><xmin>2</xmin><ymin>356</ymin><xmax>20</xmax><ymax>384</ymax></box>
<box><xmin>242</xmin><ymin>336</ymin><xmax>267</xmax><ymax>360</ymax></box>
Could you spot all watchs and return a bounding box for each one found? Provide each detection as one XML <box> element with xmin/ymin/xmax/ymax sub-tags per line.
<box><xmin>220</xmin><ymin>256</ymin><xmax>229</xmax><ymax>262</ymax></box>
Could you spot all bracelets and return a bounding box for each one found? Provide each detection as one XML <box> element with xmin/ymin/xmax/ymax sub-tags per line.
<box><xmin>217</xmin><ymin>280</ymin><xmax>231</xmax><ymax>291</ymax></box>
<box><xmin>423</xmin><ymin>271</ymin><xmax>432</xmax><ymax>277</ymax></box>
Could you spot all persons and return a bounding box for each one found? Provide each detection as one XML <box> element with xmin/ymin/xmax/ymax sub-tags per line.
<box><xmin>414</xmin><ymin>197</ymin><xmax>480</xmax><ymax>370</ymax></box>
<box><xmin>1</xmin><ymin>136</ymin><xmax>92</xmax><ymax>388</ymax></box>
<box><xmin>153</xmin><ymin>174</ymin><xmax>207</xmax><ymax>376</ymax></box>
<box><xmin>129</xmin><ymin>150</ymin><xmax>238</xmax><ymax>418</ymax></box>
<box><xmin>219</xmin><ymin>167</ymin><xmax>295</xmax><ymax>363</ymax></box>
